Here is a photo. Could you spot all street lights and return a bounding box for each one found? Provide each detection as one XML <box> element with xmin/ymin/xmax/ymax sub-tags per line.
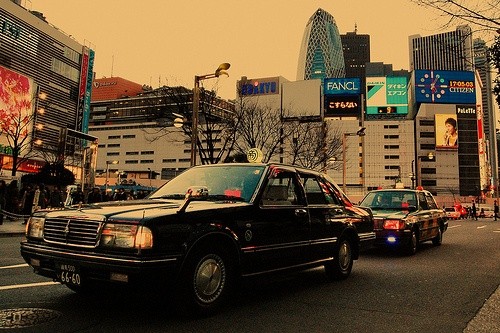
<box><xmin>189</xmin><ymin>62</ymin><xmax>232</xmax><ymax>167</ymax></box>
<box><xmin>341</xmin><ymin>131</ymin><xmax>366</xmax><ymax>194</ymax></box>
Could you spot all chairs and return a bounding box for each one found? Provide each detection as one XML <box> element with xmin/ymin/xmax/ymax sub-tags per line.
<box><xmin>263</xmin><ymin>185</ymin><xmax>291</xmax><ymax>205</ymax></box>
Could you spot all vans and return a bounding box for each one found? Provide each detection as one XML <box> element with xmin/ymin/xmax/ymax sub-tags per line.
<box><xmin>476</xmin><ymin>207</ymin><xmax>495</xmax><ymax>217</ymax></box>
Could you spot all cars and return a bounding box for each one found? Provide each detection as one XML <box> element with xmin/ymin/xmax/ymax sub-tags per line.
<box><xmin>356</xmin><ymin>188</ymin><xmax>449</xmax><ymax>256</ymax></box>
<box><xmin>19</xmin><ymin>162</ymin><xmax>379</xmax><ymax>317</ymax></box>
<box><xmin>444</xmin><ymin>207</ymin><xmax>461</xmax><ymax>220</ymax></box>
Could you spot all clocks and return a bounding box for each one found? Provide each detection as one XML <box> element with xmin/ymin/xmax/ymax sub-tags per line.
<box><xmin>416</xmin><ymin>70</ymin><xmax>449</xmax><ymax>103</ymax></box>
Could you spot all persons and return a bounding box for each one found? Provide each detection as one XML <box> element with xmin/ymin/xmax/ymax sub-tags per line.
<box><xmin>0</xmin><ymin>180</ymin><xmax>152</xmax><ymax>226</ymax></box>
<box><xmin>472</xmin><ymin>200</ymin><xmax>477</xmax><ymax>221</ymax></box>
<box><xmin>494</xmin><ymin>203</ymin><xmax>500</xmax><ymax>221</ymax></box>
<box><xmin>444</xmin><ymin>118</ymin><xmax>457</xmax><ymax>146</ymax></box>
